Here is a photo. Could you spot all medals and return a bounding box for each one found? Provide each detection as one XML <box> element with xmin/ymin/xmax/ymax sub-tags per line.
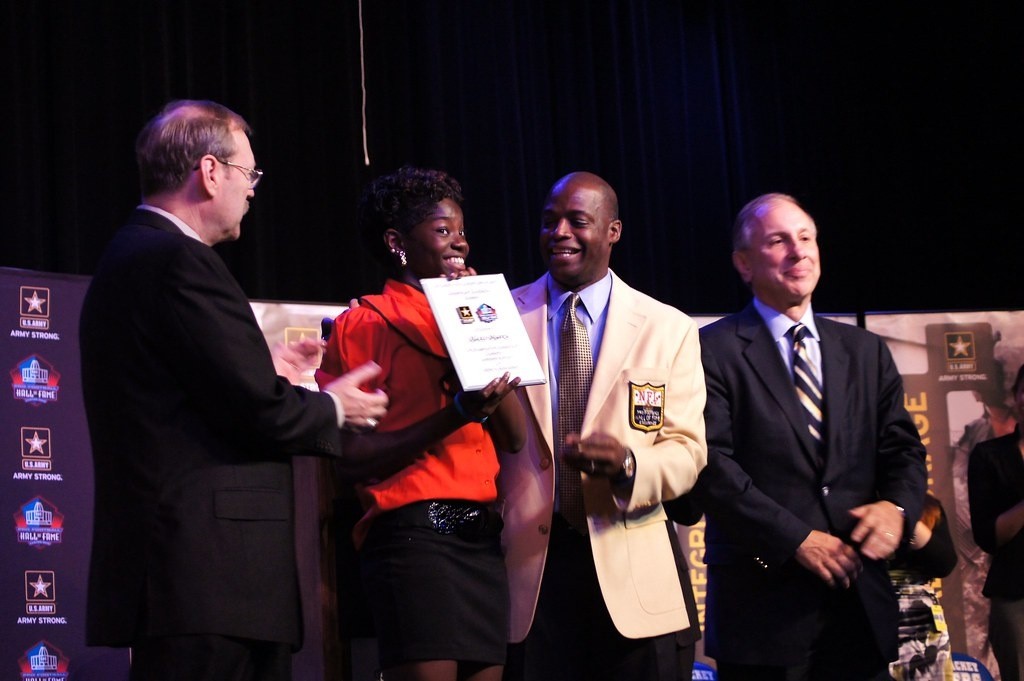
<box><xmin>457</xmin><ymin>307</ymin><xmax>474</xmax><ymax>323</ymax></box>
<box><xmin>476</xmin><ymin>304</ymin><xmax>497</xmax><ymax>323</ymax></box>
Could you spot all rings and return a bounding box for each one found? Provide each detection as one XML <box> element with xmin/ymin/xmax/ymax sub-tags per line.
<box><xmin>366</xmin><ymin>418</ymin><xmax>376</xmax><ymax>428</ymax></box>
<box><xmin>885</xmin><ymin>532</ymin><xmax>894</xmax><ymax>537</ymax></box>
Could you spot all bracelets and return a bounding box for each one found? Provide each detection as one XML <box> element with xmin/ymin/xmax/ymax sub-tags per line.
<box><xmin>895</xmin><ymin>506</ymin><xmax>904</xmax><ymax>512</ymax></box>
<box><xmin>453</xmin><ymin>390</ymin><xmax>488</xmax><ymax>423</ymax></box>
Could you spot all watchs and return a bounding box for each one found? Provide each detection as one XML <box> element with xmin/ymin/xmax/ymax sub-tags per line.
<box><xmin>622</xmin><ymin>447</ymin><xmax>635</xmax><ymax>477</ymax></box>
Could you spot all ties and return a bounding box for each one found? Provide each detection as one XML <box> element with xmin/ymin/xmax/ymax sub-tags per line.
<box><xmin>557</xmin><ymin>293</ymin><xmax>588</xmax><ymax>530</ymax></box>
<box><xmin>789</xmin><ymin>323</ymin><xmax>824</xmax><ymax>460</ymax></box>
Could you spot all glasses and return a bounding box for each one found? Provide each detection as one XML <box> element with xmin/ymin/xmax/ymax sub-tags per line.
<box><xmin>193</xmin><ymin>159</ymin><xmax>263</xmax><ymax>189</ymax></box>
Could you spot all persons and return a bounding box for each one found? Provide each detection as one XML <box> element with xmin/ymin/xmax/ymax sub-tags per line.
<box><xmin>486</xmin><ymin>172</ymin><xmax>707</xmax><ymax>681</ymax></box>
<box><xmin>316</xmin><ymin>169</ymin><xmax>530</xmax><ymax>681</ymax></box>
<box><xmin>667</xmin><ymin>191</ymin><xmax>1024</xmax><ymax>681</ymax></box>
<box><xmin>77</xmin><ymin>98</ymin><xmax>389</xmax><ymax>681</ymax></box>
